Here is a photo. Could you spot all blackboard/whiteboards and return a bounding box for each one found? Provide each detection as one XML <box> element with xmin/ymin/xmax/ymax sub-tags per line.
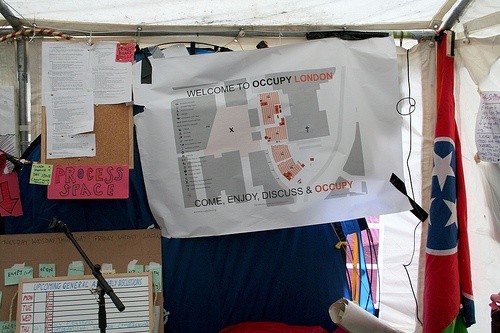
<box><xmin>12</xmin><ymin>273</ymin><xmax>155</xmax><ymax>332</ymax></box>
